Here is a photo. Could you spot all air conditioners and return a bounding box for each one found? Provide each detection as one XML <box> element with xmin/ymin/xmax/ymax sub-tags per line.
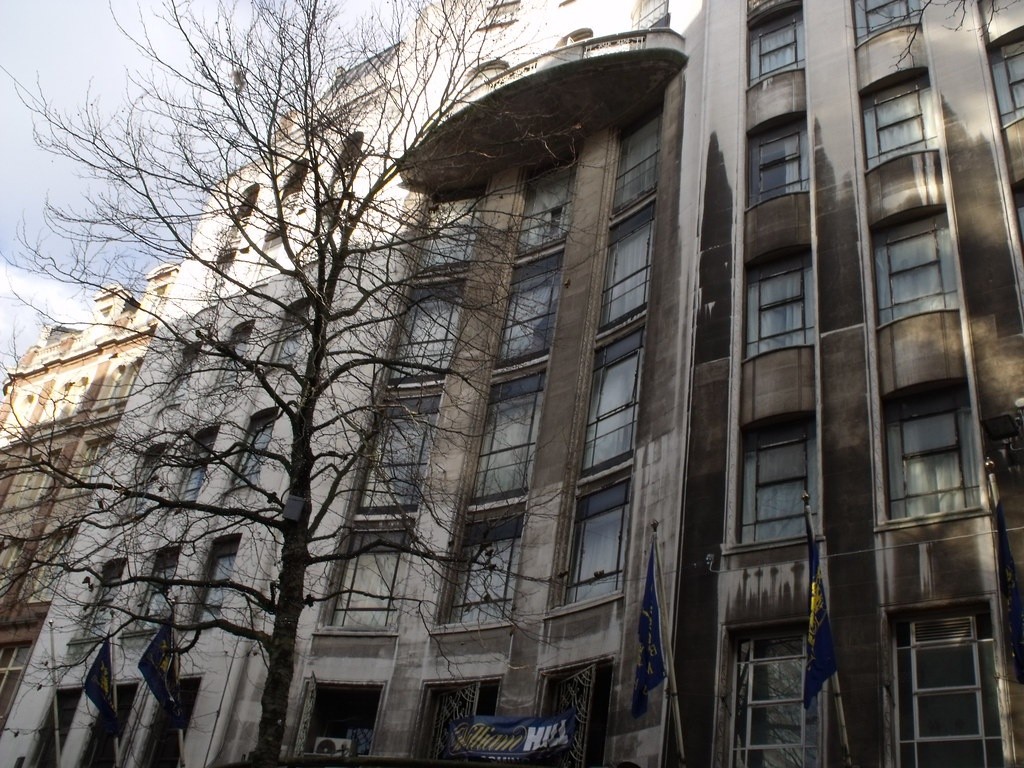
<box><xmin>314</xmin><ymin>737</ymin><xmax>352</xmax><ymax>758</ymax></box>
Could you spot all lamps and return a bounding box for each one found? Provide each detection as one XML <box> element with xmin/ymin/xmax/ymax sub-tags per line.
<box><xmin>81</xmin><ymin>576</ymin><xmax>93</xmax><ymax>592</ymax></box>
<box><xmin>97</xmin><ymin>500</ymin><xmax>104</xmax><ymax>510</ymax></box>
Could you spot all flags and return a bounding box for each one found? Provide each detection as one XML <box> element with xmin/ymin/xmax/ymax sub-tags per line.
<box><xmin>804</xmin><ymin>528</ymin><xmax>838</xmax><ymax>707</ymax></box>
<box><xmin>633</xmin><ymin>542</ymin><xmax>666</xmax><ymax>720</ymax></box>
<box><xmin>995</xmin><ymin>499</ymin><xmax>1024</xmax><ymax>684</ymax></box>
<box><xmin>84</xmin><ymin>636</ymin><xmax>121</xmax><ymax>737</ymax></box>
<box><xmin>138</xmin><ymin>612</ymin><xmax>184</xmax><ymax>730</ymax></box>
